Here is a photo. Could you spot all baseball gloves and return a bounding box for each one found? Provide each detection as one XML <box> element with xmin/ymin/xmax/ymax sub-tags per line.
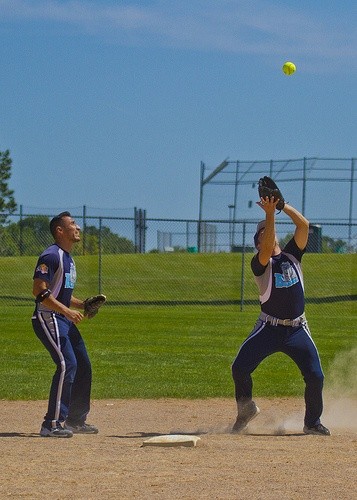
<box><xmin>258</xmin><ymin>176</ymin><xmax>285</xmax><ymax>211</ymax></box>
<box><xmin>84</xmin><ymin>294</ymin><xmax>106</xmax><ymax>318</ymax></box>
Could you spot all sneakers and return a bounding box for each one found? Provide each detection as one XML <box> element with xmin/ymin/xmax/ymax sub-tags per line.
<box><xmin>303</xmin><ymin>424</ymin><xmax>330</xmax><ymax>436</ymax></box>
<box><xmin>40</xmin><ymin>424</ymin><xmax>74</xmax><ymax>438</ymax></box>
<box><xmin>64</xmin><ymin>422</ymin><xmax>99</xmax><ymax>433</ymax></box>
<box><xmin>232</xmin><ymin>406</ymin><xmax>260</xmax><ymax>433</ymax></box>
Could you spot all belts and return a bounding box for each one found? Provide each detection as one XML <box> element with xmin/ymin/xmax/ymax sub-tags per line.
<box><xmin>260</xmin><ymin>312</ymin><xmax>305</xmax><ymax>326</ymax></box>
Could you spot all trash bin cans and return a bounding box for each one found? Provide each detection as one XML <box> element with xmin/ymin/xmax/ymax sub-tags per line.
<box><xmin>305</xmin><ymin>224</ymin><xmax>322</xmax><ymax>253</ymax></box>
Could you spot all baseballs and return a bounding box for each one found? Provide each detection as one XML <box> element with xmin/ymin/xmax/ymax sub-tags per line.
<box><xmin>282</xmin><ymin>62</ymin><xmax>296</xmax><ymax>75</ymax></box>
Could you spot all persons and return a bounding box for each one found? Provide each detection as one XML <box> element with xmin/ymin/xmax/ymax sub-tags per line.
<box><xmin>32</xmin><ymin>211</ymin><xmax>98</xmax><ymax>438</ymax></box>
<box><xmin>232</xmin><ymin>195</ymin><xmax>331</xmax><ymax>436</ymax></box>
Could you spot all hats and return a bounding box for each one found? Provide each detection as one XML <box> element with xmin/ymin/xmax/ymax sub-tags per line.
<box><xmin>253</xmin><ymin>220</ymin><xmax>265</xmax><ymax>246</ymax></box>
